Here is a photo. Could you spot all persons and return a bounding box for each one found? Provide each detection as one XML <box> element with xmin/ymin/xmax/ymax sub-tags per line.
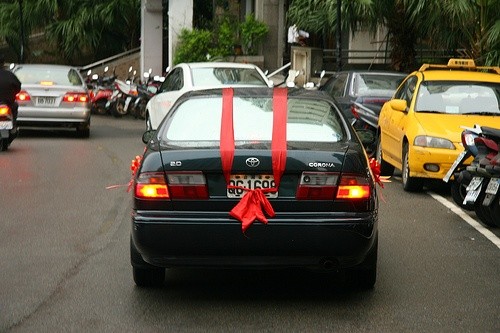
<box><xmin>0</xmin><ymin>59</ymin><xmax>21</xmax><ymax>123</ymax></box>
<box><xmin>283</xmin><ymin>19</ymin><xmax>306</xmax><ymax>74</ymax></box>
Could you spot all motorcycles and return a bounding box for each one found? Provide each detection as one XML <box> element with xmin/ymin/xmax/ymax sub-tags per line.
<box><xmin>134</xmin><ymin>73</ymin><xmax>171</xmax><ymax>120</ymax></box>
<box><xmin>349</xmin><ymin>101</ymin><xmax>382</xmax><ymax>155</ymax></box>
<box><xmin>85</xmin><ymin>66</ymin><xmax>118</xmax><ymax>89</ymax></box>
<box><xmin>0</xmin><ymin>104</ymin><xmax>18</xmax><ymax>150</ymax></box>
<box><xmin>443</xmin><ymin>124</ymin><xmax>500</xmax><ymax>209</ymax></box>
<box><xmin>122</xmin><ymin>68</ymin><xmax>154</xmax><ymax>116</ymax></box>
<box><xmin>106</xmin><ymin>66</ymin><xmax>137</xmax><ymax>116</ymax></box>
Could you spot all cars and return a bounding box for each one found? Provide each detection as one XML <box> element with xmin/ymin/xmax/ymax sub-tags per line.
<box><xmin>130</xmin><ymin>87</ymin><xmax>379</xmax><ymax>293</ymax></box>
<box><xmin>145</xmin><ymin>62</ymin><xmax>274</xmax><ymax>131</ymax></box>
<box><xmin>376</xmin><ymin>59</ymin><xmax>500</xmax><ymax>191</ymax></box>
<box><xmin>12</xmin><ymin>64</ymin><xmax>89</xmax><ymax>133</ymax></box>
<box><xmin>321</xmin><ymin>70</ymin><xmax>409</xmax><ymax>121</ymax></box>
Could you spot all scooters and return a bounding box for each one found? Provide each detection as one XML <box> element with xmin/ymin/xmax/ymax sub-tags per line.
<box><xmin>302</xmin><ymin>70</ymin><xmax>326</xmax><ymax>89</ymax></box>
<box><xmin>92</xmin><ymin>66</ymin><xmax>133</xmax><ymax>114</ymax></box>
